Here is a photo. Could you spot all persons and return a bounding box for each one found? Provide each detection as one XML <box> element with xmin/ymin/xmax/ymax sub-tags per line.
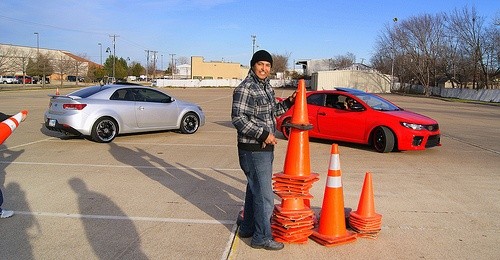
<box><xmin>0</xmin><ymin>111</ymin><xmax>27</xmax><ymax>218</ymax></box>
<box><xmin>345</xmin><ymin>98</ymin><xmax>354</xmax><ymax>110</ymax></box>
<box><xmin>231</xmin><ymin>50</ymin><xmax>296</xmax><ymax>250</ymax></box>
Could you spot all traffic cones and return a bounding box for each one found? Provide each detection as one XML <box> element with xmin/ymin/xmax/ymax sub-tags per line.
<box><xmin>0</xmin><ymin>110</ymin><xmax>29</xmax><ymax>145</ymax></box>
<box><xmin>272</xmin><ymin>79</ymin><xmax>383</xmax><ymax>248</ymax></box>
<box><xmin>56</xmin><ymin>87</ymin><xmax>60</xmax><ymax>95</ymax></box>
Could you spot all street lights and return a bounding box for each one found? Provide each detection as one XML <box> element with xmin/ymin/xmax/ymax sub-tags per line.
<box><xmin>98</xmin><ymin>43</ymin><xmax>102</xmax><ymax>65</ymax></box>
<box><xmin>34</xmin><ymin>32</ymin><xmax>39</xmax><ymax>64</ymax></box>
<box><xmin>390</xmin><ymin>17</ymin><xmax>397</xmax><ymax>91</ymax></box>
<box><xmin>106</xmin><ymin>35</ymin><xmax>116</xmax><ymax>82</ymax></box>
<box><xmin>251</xmin><ymin>34</ymin><xmax>259</xmax><ymax>54</ymax></box>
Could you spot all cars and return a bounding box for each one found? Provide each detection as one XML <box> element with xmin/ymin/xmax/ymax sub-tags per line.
<box><xmin>43</xmin><ymin>82</ymin><xmax>206</xmax><ymax>144</ymax></box>
<box><xmin>0</xmin><ymin>75</ymin><xmax>85</xmax><ymax>84</ymax></box>
<box><xmin>275</xmin><ymin>86</ymin><xmax>442</xmax><ymax>155</ymax></box>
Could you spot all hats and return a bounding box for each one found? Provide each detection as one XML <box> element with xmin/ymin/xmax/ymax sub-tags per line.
<box><xmin>250</xmin><ymin>50</ymin><xmax>273</xmax><ymax>68</ymax></box>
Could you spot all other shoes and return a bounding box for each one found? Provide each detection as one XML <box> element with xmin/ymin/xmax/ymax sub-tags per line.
<box><xmin>238</xmin><ymin>232</ymin><xmax>254</xmax><ymax>238</ymax></box>
<box><xmin>0</xmin><ymin>209</ymin><xmax>14</xmax><ymax>218</ymax></box>
<box><xmin>251</xmin><ymin>239</ymin><xmax>284</xmax><ymax>250</ymax></box>
